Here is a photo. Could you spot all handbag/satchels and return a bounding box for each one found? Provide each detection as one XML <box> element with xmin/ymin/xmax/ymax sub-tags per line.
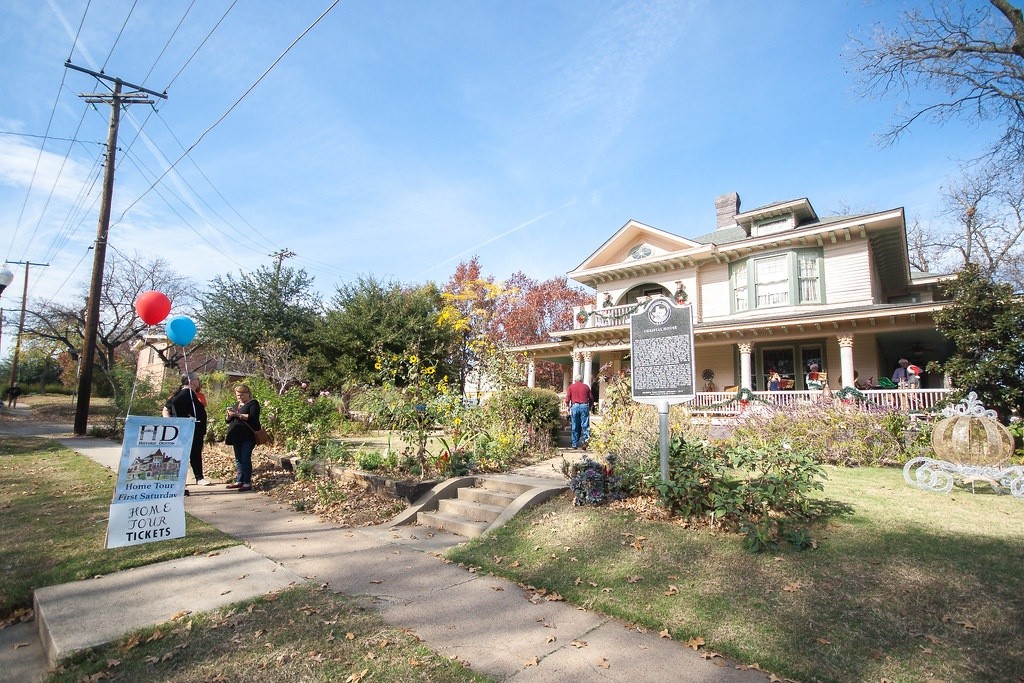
<box><xmin>255</xmin><ymin>429</ymin><xmax>270</xmax><ymax>447</ymax></box>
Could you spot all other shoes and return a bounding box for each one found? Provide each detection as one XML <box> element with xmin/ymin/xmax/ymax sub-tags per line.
<box><xmin>238</xmin><ymin>483</ymin><xmax>252</xmax><ymax>492</ymax></box>
<box><xmin>196</xmin><ymin>479</ymin><xmax>211</xmax><ymax>486</ymax></box>
<box><xmin>572</xmin><ymin>447</ymin><xmax>577</xmax><ymax>450</ymax></box>
<box><xmin>582</xmin><ymin>444</ymin><xmax>586</xmax><ymax>450</ymax></box>
<box><xmin>226</xmin><ymin>481</ymin><xmax>244</xmax><ymax>488</ymax></box>
<box><xmin>183</xmin><ymin>490</ymin><xmax>190</xmax><ymax>498</ymax></box>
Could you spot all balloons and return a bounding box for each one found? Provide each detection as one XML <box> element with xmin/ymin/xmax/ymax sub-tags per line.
<box><xmin>135</xmin><ymin>290</ymin><xmax>171</xmax><ymax>326</ymax></box>
<box><xmin>165</xmin><ymin>315</ymin><xmax>197</xmax><ymax>347</ymax></box>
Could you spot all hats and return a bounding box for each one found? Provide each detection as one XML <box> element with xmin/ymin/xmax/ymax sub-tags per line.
<box><xmin>907</xmin><ymin>365</ymin><xmax>922</xmax><ymax>379</ymax></box>
<box><xmin>810</xmin><ymin>361</ymin><xmax>818</xmax><ymax>370</ymax></box>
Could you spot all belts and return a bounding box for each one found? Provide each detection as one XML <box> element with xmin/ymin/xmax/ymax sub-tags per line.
<box><xmin>574</xmin><ymin>402</ymin><xmax>589</xmax><ymax>404</ymax></box>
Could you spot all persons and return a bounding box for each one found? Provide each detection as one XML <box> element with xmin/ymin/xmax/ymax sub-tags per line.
<box><xmin>767</xmin><ymin>368</ymin><xmax>781</xmax><ymax>391</ymax></box>
<box><xmin>854</xmin><ymin>369</ymin><xmax>872</xmax><ymax>390</ymax></box>
<box><xmin>163</xmin><ymin>372</ymin><xmax>212</xmax><ymax>487</ymax></box>
<box><xmin>891</xmin><ymin>358</ymin><xmax>921</xmax><ymax>389</ymax></box>
<box><xmin>224</xmin><ymin>385</ymin><xmax>261</xmax><ymax>491</ymax></box>
<box><xmin>7</xmin><ymin>382</ymin><xmax>22</xmax><ymax>409</ymax></box>
<box><xmin>806</xmin><ymin>363</ymin><xmax>825</xmax><ymax>400</ymax></box>
<box><xmin>566</xmin><ymin>374</ymin><xmax>594</xmax><ymax>450</ymax></box>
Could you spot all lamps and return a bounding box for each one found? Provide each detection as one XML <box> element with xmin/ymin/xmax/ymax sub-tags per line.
<box><xmin>675</xmin><ymin>280</ymin><xmax>682</xmax><ymax>292</ymax></box>
<box><xmin>602</xmin><ymin>289</ymin><xmax>609</xmax><ymax>301</ymax></box>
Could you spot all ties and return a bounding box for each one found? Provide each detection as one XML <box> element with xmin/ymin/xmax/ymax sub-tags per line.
<box><xmin>904</xmin><ymin>368</ymin><xmax>908</xmax><ymax>381</ymax></box>
